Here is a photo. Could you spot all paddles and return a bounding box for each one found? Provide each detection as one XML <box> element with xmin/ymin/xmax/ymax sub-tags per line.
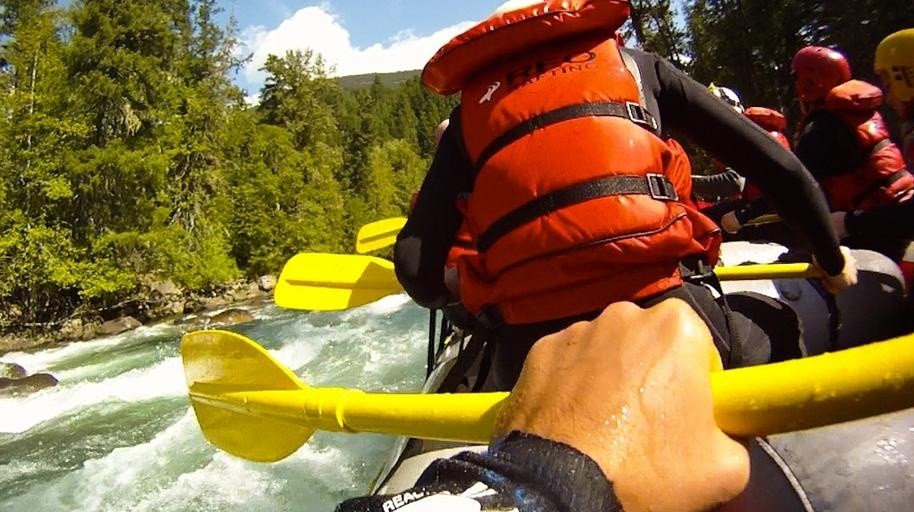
<box><xmin>356</xmin><ymin>216</ymin><xmax>408</xmax><ymax>253</ymax></box>
<box><xmin>182</xmin><ymin>329</ymin><xmax>914</xmax><ymax>464</ymax></box>
<box><xmin>273</xmin><ymin>252</ymin><xmax>827</xmax><ymax>312</ymax></box>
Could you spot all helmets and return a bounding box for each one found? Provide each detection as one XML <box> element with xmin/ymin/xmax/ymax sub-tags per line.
<box><xmin>790</xmin><ymin>44</ymin><xmax>853</xmax><ymax>102</ymax></box>
<box><xmin>872</xmin><ymin>26</ymin><xmax>913</xmax><ymax>102</ymax></box>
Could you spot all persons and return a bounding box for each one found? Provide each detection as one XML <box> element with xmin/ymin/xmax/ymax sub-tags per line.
<box><xmin>700</xmin><ymin>238</ymin><xmax>906</xmax><ymax>366</ymax></box>
<box><xmin>333</xmin><ymin>296</ymin><xmax>752</xmax><ymax>512</ymax></box>
<box><xmin>874</xmin><ymin>26</ymin><xmax>914</xmax><ymax>174</ymax></box>
<box><xmin>394</xmin><ymin>0</ymin><xmax>859</xmax><ymax>389</ymax></box>
<box><xmin>722</xmin><ymin>43</ymin><xmax>912</xmax><ymax>268</ymax></box>
<box><xmin>686</xmin><ymin>87</ymin><xmax>790</xmax><ymax>232</ymax></box>
